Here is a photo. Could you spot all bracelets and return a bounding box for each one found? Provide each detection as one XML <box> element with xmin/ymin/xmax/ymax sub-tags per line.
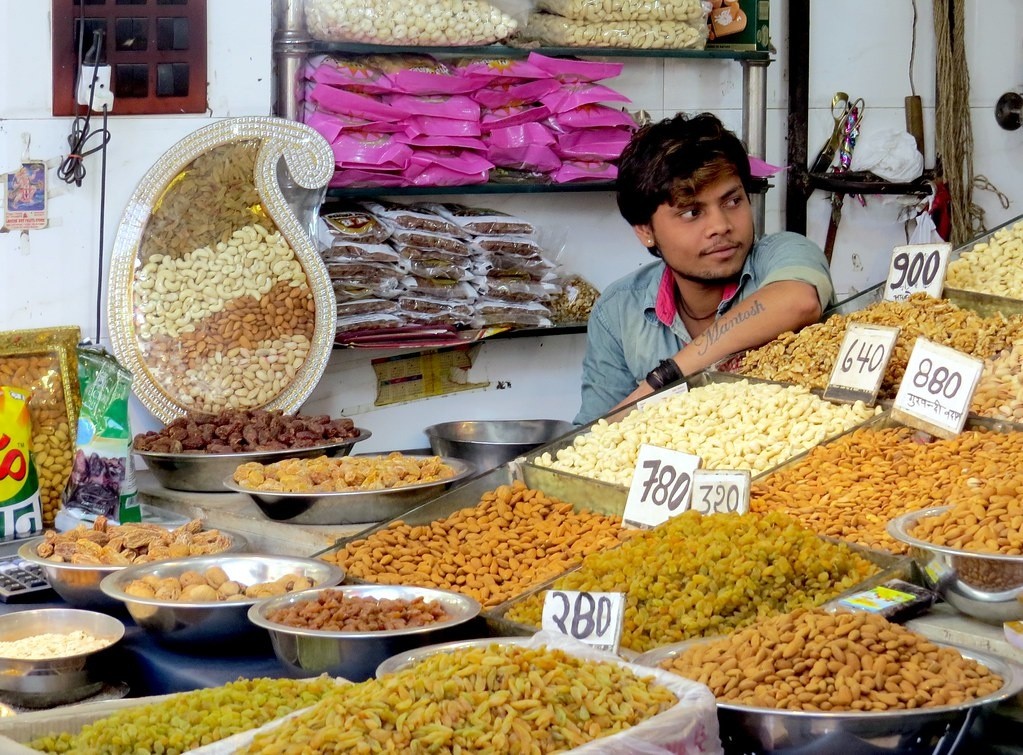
<box><xmin>646</xmin><ymin>359</ymin><xmax>684</xmax><ymax>390</ymax></box>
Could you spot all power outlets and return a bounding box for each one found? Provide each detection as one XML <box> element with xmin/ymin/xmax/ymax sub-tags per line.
<box><xmin>75</xmin><ymin>63</ymin><xmax>111</xmax><ymax>105</ymax></box>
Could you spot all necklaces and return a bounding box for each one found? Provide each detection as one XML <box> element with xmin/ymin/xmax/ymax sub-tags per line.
<box><xmin>678</xmin><ymin>296</ymin><xmax>718</xmax><ymax>320</ymax></box>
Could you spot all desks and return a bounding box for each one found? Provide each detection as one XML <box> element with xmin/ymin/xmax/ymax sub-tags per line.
<box><xmin>134</xmin><ymin>471</ymin><xmax>379</xmax><ymax>556</ymax></box>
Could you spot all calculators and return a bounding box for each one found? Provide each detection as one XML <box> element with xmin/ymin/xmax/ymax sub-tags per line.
<box><xmin>1</xmin><ymin>534</ymin><xmax>55</xmax><ymax>605</ymax></box>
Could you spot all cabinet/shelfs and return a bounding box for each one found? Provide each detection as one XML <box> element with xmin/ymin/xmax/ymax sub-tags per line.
<box><xmin>269</xmin><ymin>0</ymin><xmax>777</xmax><ymax>350</ymax></box>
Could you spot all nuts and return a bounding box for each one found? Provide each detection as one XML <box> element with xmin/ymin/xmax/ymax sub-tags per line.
<box><xmin>19</xmin><ymin>646</ymin><xmax>681</xmax><ymax>755</ymax></box>
<box><xmin>321</xmin><ymin>219</ymin><xmax>1023</xmax><ymax>716</ymax></box>
<box><xmin>125</xmin><ymin>564</ymin><xmax>316</xmax><ymax>604</ymax></box>
<box><xmin>128</xmin><ymin>139</ymin><xmax>315</xmax><ymax>411</ymax></box>
<box><xmin>0</xmin><ymin>354</ymin><xmax>75</xmax><ymax>522</ymax></box>
<box><xmin>524</xmin><ymin>0</ymin><xmax>711</xmax><ymax>50</ymax></box>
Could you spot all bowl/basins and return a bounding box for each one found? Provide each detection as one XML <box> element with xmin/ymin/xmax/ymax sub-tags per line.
<box><xmin>19</xmin><ymin>525</ymin><xmax>246</xmax><ymax>604</ymax></box>
<box><xmin>887</xmin><ymin>507</ymin><xmax>1023</xmax><ymax>626</ymax></box>
<box><xmin>223</xmin><ymin>456</ymin><xmax>474</xmax><ymax>526</ymax></box>
<box><xmin>134</xmin><ymin>428</ymin><xmax>373</xmax><ymax>493</ymax></box>
<box><xmin>99</xmin><ymin>553</ymin><xmax>346</xmax><ymax>647</ymax></box>
<box><xmin>247</xmin><ymin>586</ymin><xmax>480</xmax><ymax>681</ymax></box>
<box><xmin>376</xmin><ymin>636</ymin><xmax>545</xmax><ymax>685</ymax></box>
<box><xmin>1</xmin><ymin>610</ymin><xmax>125</xmax><ymax>711</ymax></box>
<box><xmin>631</xmin><ymin>638</ymin><xmax>1023</xmax><ymax>755</ymax></box>
<box><xmin>425</xmin><ymin>419</ymin><xmax>571</xmax><ymax>488</ymax></box>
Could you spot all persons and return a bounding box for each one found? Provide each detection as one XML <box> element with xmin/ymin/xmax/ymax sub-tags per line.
<box><xmin>572</xmin><ymin>113</ymin><xmax>832</xmax><ymax>430</ymax></box>
